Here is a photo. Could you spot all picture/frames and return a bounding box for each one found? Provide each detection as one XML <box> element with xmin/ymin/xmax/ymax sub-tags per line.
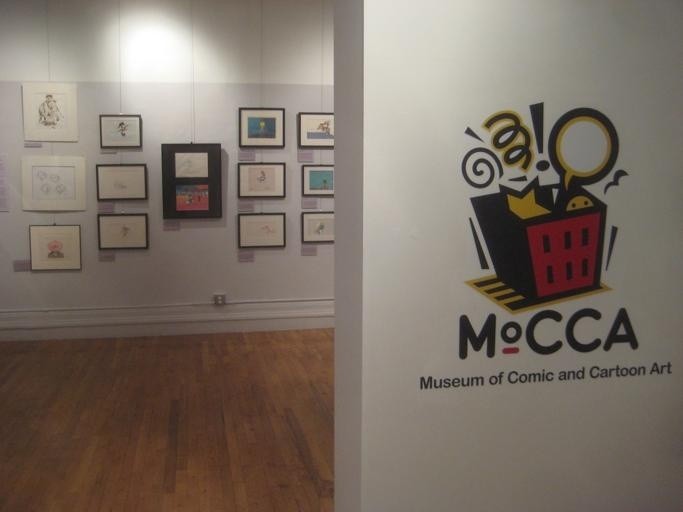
<box><xmin>300</xmin><ymin>209</ymin><xmax>334</xmax><ymax>245</ymax></box>
<box><xmin>300</xmin><ymin>163</ymin><xmax>334</xmax><ymax>198</ymax></box>
<box><xmin>96</xmin><ymin>113</ymin><xmax>143</xmax><ymax>151</ymax></box>
<box><xmin>27</xmin><ymin>224</ymin><xmax>80</xmax><ymax>270</ymax></box>
<box><xmin>95</xmin><ymin>211</ymin><xmax>149</xmax><ymax>250</ymax></box>
<box><xmin>296</xmin><ymin>111</ymin><xmax>335</xmax><ymax>150</ymax></box>
<box><xmin>17</xmin><ymin>82</ymin><xmax>90</xmax><ymax>215</ymax></box>
<box><xmin>237</xmin><ymin>106</ymin><xmax>285</xmax><ymax>150</ymax></box>
<box><xmin>93</xmin><ymin>162</ymin><xmax>149</xmax><ymax>202</ymax></box>
<box><xmin>237</xmin><ymin>211</ymin><xmax>286</xmax><ymax>250</ymax></box>
<box><xmin>235</xmin><ymin>160</ymin><xmax>287</xmax><ymax>200</ymax></box>
